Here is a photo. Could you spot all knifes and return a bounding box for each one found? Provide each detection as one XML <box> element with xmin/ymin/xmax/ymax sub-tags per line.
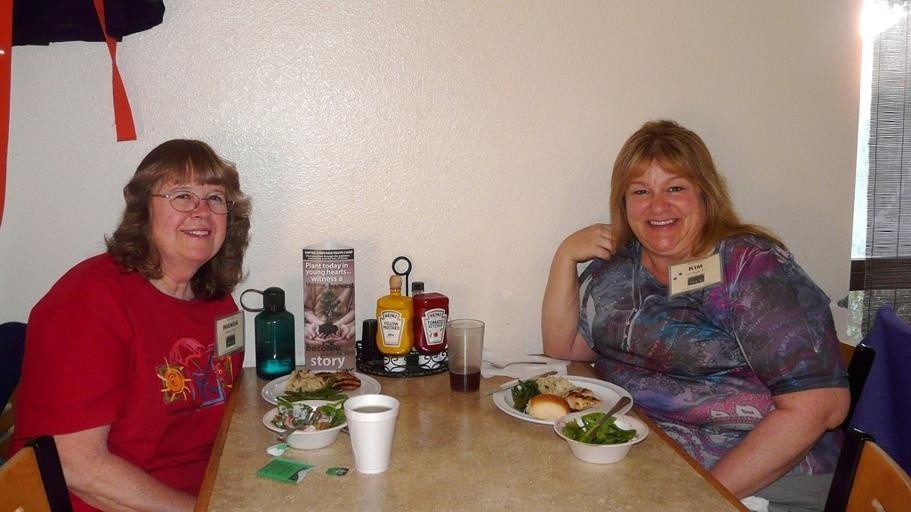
<box><xmin>484</xmin><ymin>370</ymin><xmax>558</xmax><ymax>396</ymax></box>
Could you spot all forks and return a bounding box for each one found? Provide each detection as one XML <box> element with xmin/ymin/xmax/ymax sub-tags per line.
<box><xmin>576</xmin><ymin>397</ymin><xmax>630</xmax><ymax>443</ymax></box>
<box><xmin>277</xmin><ymin>425</ymin><xmax>300</xmax><ymax>442</ymax></box>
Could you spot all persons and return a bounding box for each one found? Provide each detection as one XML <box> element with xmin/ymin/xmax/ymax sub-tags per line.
<box><xmin>541</xmin><ymin>118</ymin><xmax>852</xmax><ymax>512</ymax></box>
<box><xmin>2</xmin><ymin>138</ymin><xmax>250</xmax><ymax>512</ymax></box>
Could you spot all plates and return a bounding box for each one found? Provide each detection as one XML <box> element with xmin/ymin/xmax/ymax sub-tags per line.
<box><xmin>261</xmin><ymin>370</ymin><xmax>382</xmax><ymax>405</ymax></box>
<box><xmin>493</xmin><ymin>373</ymin><xmax>632</xmax><ymax>424</ymax></box>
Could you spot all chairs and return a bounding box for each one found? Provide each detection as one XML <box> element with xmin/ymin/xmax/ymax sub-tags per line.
<box><xmin>824</xmin><ymin>427</ymin><xmax>911</xmax><ymax>512</ymax></box>
<box><xmin>836</xmin><ymin>338</ymin><xmax>874</xmax><ymax>398</ymax></box>
<box><xmin>0</xmin><ymin>434</ymin><xmax>75</xmax><ymax>512</ymax></box>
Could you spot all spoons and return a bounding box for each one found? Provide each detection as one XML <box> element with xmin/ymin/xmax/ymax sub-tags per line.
<box><xmin>483</xmin><ymin>360</ymin><xmax>547</xmax><ymax>369</ymax></box>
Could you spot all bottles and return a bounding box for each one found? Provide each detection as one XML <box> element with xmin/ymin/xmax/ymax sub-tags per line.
<box><xmin>411</xmin><ymin>282</ymin><xmax>425</xmax><ymax>297</ymax></box>
<box><xmin>375</xmin><ymin>275</ymin><xmax>413</xmax><ymax>355</ymax></box>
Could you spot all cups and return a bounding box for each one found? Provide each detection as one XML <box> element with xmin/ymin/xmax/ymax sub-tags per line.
<box><xmin>344</xmin><ymin>395</ymin><xmax>399</xmax><ymax>473</ymax></box>
<box><xmin>446</xmin><ymin>320</ymin><xmax>486</xmax><ymax>392</ymax></box>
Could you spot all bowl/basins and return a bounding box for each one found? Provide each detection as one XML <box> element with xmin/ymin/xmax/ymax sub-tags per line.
<box><xmin>553</xmin><ymin>410</ymin><xmax>649</xmax><ymax>464</ymax></box>
<box><xmin>263</xmin><ymin>400</ymin><xmax>347</xmax><ymax>450</ymax></box>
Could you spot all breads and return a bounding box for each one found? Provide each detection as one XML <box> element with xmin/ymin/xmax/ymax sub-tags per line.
<box><xmin>526</xmin><ymin>394</ymin><xmax>571</xmax><ymax>421</ymax></box>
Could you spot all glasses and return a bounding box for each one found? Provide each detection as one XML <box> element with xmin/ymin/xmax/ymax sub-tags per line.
<box><xmin>152</xmin><ymin>189</ymin><xmax>235</xmax><ymax>215</ymax></box>
